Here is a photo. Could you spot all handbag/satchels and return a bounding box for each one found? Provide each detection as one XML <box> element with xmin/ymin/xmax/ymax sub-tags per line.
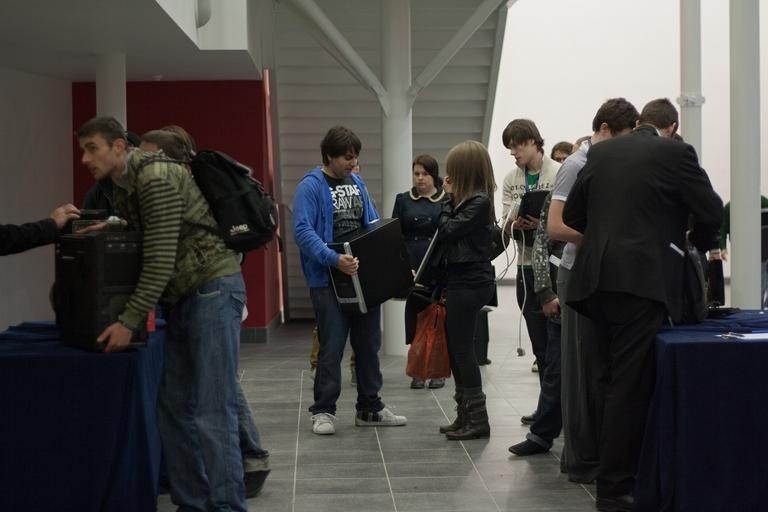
<box><xmin>453</xmin><ymin>193</ymin><xmax>510</xmax><ymax>262</ymax></box>
<box><xmin>683</xmin><ymin>246</ymin><xmax>708</xmax><ymax>326</ymax></box>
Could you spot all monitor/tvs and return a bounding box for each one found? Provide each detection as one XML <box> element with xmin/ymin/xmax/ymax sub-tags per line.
<box><xmin>414</xmin><ymin>226</ymin><xmax>442</xmax><ymax>296</ymax></box>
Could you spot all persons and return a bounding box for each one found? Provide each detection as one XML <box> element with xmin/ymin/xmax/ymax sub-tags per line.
<box><xmin>78</xmin><ymin>126</ymin><xmax>271</xmax><ymax>499</ymax></box>
<box><xmin>547</xmin><ymin>136</ymin><xmax>574</xmax><ymax>164</ymax></box>
<box><xmin>436</xmin><ymin>141</ymin><xmax>505</xmax><ymax>440</ymax></box>
<box><xmin>561</xmin><ymin>99</ymin><xmax>722</xmax><ymax>512</ymax></box>
<box><xmin>292</xmin><ymin>126</ymin><xmax>407</xmax><ymax>435</ymax></box>
<box><xmin>574</xmin><ymin>136</ymin><xmax>594</xmax><ymax>155</ymax></box>
<box><xmin>501</xmin><ymin>119</ymin><xmax>566</xmax><ymax>454</ymax></box>
<box><xmin>722</xmin><ymin>194</ymin><xmax>768</xmax><ymax>310</ymax></box>
<box><xmin>391</xmin><ymin>155</ymin><xmax>450</xmax><ymax>388</ymax></box>
<box><xmin>0</xmin><ymin>203</ymin><xmax>81</xmax><ymax>257</ymax></box>
<box><xmin>76</xmin><ymin>118</ymin><xmax>244</xmax><ymax>512</ymax></box>
<box><xmin>545</xmin><ymin>99</ymin><xmax>640</xmax><ymax>477</ymax></box>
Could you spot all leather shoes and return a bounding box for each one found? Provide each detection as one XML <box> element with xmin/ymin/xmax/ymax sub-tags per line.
<box><xmin>509</xmin><ymin>440</ymin><xmax>551</xmax><ymax>455</ymax></box>
<box><xmin>243</xmin><ymin>471</ymin><xmax>270</xmax><ymax>498</ymax></box>
<box><xmin>429</xmin><ymin>378</ymin><xmax>445</xmax><ymax>388</ymax></box>
<box><xmin>596</xmin><ymin>489</ymin><xmax>635</xmax><ymax>512</ymax></box>
<box><xmin>411</xmin><ymin>377</ymin><xmax>425</xmax><ymax>388</ymax></box>
<box><xmin>522</xmin><ymin>409</ymin><xmax>537</xmax><ymax>424</ymax></box>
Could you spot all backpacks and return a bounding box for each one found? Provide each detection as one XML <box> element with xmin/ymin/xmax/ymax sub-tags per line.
<box><xmin>132</xmin><ymin>150</ymin><xmax>286</xmax><ymax>257</ymax></box>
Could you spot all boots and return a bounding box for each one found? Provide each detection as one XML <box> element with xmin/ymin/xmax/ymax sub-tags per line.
<box><xmin>446</xmin><ymin>393</ymin><xmax>490</xmax><ymax>440</ymax></box>
<box><xmin>440</xmin><ymin>391</ymin><xmax>465</xmax><ymax>433</ymax></box>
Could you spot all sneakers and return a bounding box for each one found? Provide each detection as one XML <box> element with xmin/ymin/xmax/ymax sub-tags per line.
<box><xmin>311</xmin><ymin>413</ymin><xmax>339</xmax><ymax>435</ymax></box>
<box><xmin>530</xmin><ymin>359</ymin><xmax>541</xmax><ymax>372</ymax></box>
<box><xmin>354</xmin><ymin>408</ymin><xmax>406</xmax><ymax>427</ymax></box>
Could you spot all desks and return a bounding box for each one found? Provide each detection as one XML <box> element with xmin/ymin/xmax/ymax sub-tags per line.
<box><xmin>0</xmin><ymin>319</ymin><xmax>163</xmax><ymax>512</ymax></box>
<box><xmin>635</xmin><ymin>307</ymin><xmax>768</xmax><ymax>511</ymax></box>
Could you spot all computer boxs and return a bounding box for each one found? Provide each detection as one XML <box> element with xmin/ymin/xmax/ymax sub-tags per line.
<box><xmin>325</xmin><ymin>216</ymin><xmax>413</xmax><ymax>318</ymax></box>
<box><xmin>53</xmin><ymin>232</ymin><xmax>150</xmax><ymax>349</ymax></box>
